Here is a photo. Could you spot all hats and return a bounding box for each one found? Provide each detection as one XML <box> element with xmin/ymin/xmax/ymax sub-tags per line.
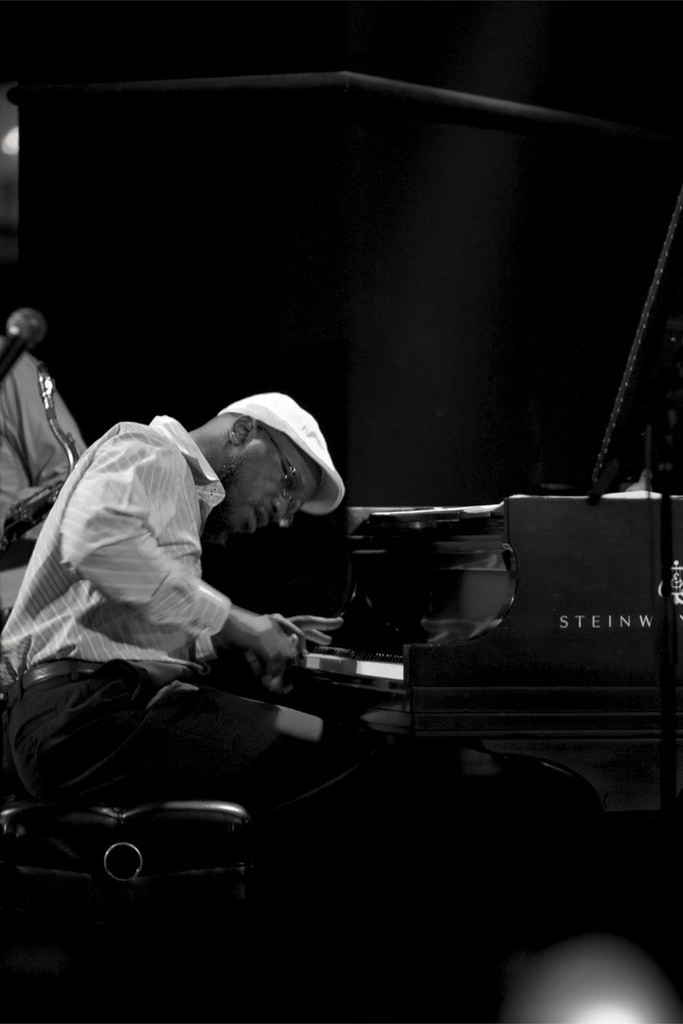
<box><xmin>217</xmin><ymin>392</ymin><xmax>345</xmax><ymax>516</ymax></box>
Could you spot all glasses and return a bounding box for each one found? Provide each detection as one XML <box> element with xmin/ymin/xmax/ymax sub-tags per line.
<box><xmin>253</xmin><ymin>424</ymin><xmax>300</xmax><ymax>531</ymax></box>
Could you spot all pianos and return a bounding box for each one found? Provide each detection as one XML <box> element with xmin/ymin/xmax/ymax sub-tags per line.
<box><xmin>183</xmin><ymin>490</ymin><xmax>682</xmax><ymax>821</ymax></box>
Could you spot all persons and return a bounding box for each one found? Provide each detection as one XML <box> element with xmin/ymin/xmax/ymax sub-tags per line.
<box><xmin>0</xmin><ymin>338</ymin><xmax>88</xmax><ymax>615</ymax></box>
<box><xmin>0</xmin><ymin>389</ymin><xmax>361</xmax><ymax>987</ymax></box>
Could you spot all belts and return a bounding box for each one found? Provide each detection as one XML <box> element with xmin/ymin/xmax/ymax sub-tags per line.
<box><xmin>5</xmin><ymin>659</ymin><xmax>104</xmax><ymax>709</ymax></box>
